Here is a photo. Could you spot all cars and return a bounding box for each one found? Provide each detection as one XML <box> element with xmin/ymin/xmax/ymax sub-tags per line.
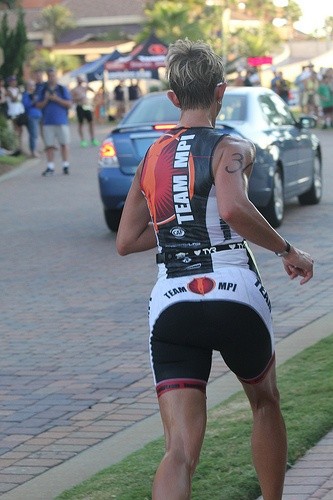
<box><xmin>97</xmin><ymin>87</ymin><xmax>323</xmax><ymax>234</ymax></box>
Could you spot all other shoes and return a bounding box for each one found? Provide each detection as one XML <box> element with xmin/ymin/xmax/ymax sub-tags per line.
<box><xmin>91</xmin><ymin>140</ymin><xmax>99</xmax><ymax>147</ymax></box>
<box><xmin>81</xmin><ymin>141</ymin><xmax>88</xmax><ymax>146</ymax></box>
<box><xmin>62</xmin><ymin>167</ymin><xmax>68</xmax><ymax>174</ymax></box>
<box><xmin>42</xmin><ymin>168</ymin><xmax>53</xmax><ymax>176</ymax></box>
